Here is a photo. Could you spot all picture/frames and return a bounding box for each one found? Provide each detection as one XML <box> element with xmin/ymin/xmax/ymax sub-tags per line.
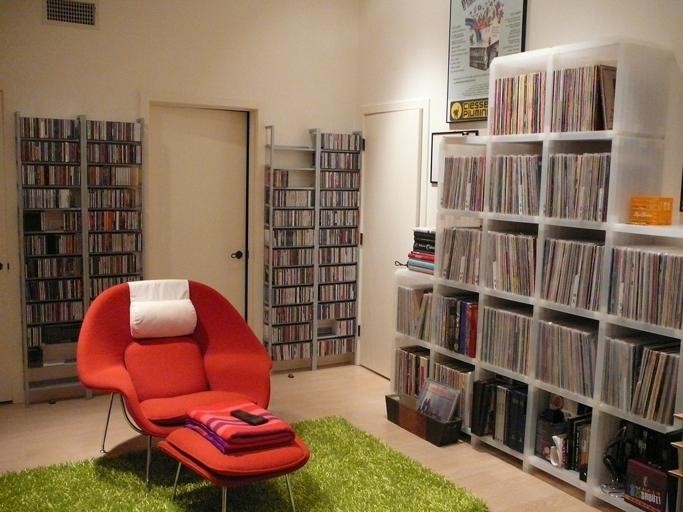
<box><xmin>428</xmin><ymin>129</ymin><xmax>479</xmax><ymax>185</ymax></box>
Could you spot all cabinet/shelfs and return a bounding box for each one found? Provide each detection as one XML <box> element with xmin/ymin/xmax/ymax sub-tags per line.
<box><xmin>263</xmin><ymin>124</ymin><xmax>364</xmax><ymax>374</ymax></box>
<box><xmin>12</xmin><ymin>108</ymin><xmax>148</xmax><ymax>410</ymax></box>
<box><xmin>389</xmin><ymin>36</ymin><xmax>682</xmax><ymax>511</ymax></box>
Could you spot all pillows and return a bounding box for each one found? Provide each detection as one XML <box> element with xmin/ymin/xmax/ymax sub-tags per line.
<box><xmin>127</xmin><ymin>279</ymin><xmax>196</xmax><ymax>339</ymax></box>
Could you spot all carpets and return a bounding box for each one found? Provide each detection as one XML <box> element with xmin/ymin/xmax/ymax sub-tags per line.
<box><xmin>0</xmin><ymin>411</ymin><xmax>489</xmax><ymax>512</ymax></box>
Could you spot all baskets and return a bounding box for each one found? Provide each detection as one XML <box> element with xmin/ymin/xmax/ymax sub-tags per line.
<box><xmin>629</xmin><ymin>196</ymin><xmax>674</xmax><ymax>225</ymax></box>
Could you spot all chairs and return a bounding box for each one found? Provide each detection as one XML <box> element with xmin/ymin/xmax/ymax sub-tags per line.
<box><xmin>74</xmin><ymin>278</ymin><xmax>273</xmax><ymax>484</ymax></box>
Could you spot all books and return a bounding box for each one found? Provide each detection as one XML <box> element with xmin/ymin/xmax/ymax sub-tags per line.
<box><xmin>489</xmin><ymin>154</ymin><xmax>541</xmax><ymax>216</ymax></box>
<box><xmin>486</xmin><ymin>231</ymin><xmax>536</xmax><ymax>297</ymax></box>
<box><xmin>492</xmin><ymin>72</ymin><xmax>546</xmax><ymax>135</ymax></box>
<box><xmin>471</xmin><ymin>307</ymin><xmax>535</xmax><ymax>455</ymax></box>
<box><xmin>441</xmin><ymin>157</ymin><xmax>486</xmax><ymax>212</ymax></box>
<box><xmin>406</xmin><ymin>227</ymin><xmax>436</xmax><ymax>274</ymax></box>
<box><xmin>542</xmin><ymin>236</ymin><xmax>604</xmax><ymax>311</ymax></box>
<box><xmin>609</xmin><ymin>246</ymin><xmax>682</xmax><ymax>329</ymax></box>
<box><xmin>395</xmin><ymin>286</ymin><xmax>479</xmax><ymax>429</ymax></box>
<box><xmin>546</xmin><ymin>152</ymin><xmax>610</xmax><ymax>222</ymax></box>
<box><xmin>535</xmin><ymin>316</ymin><xmax>682</xmax><ymax>482</ymax></box>
<box><xmin>20</xmin><ymin>117</ymin><xmax>142</xmax><ymax>348</ymax></box>
<box><xmin>439</xmin><ymin>227</ymin><xmax>482</xmax><ymax>286</ymax></box>
<box><xmin>264</xmin><ymin>133</ymin><xmax>360</xmax><ymax>361</ymax></box>
<box><xmin>551</xmin><ymin>65</ymin><xmax>615</xmax><ymax>131</ymax></box>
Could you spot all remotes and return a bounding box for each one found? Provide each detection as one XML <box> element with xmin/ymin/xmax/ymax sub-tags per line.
<box><xmin>231</xmin><ymin>410</ymin><xmax>266</xmax><ymax>425</ymax></box>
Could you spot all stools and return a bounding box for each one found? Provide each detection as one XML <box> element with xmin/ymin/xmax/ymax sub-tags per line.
<box><xmin>158</xmin><ymin>424</ymin><xmax>309</xmax><ymax>511</ymax></box>
